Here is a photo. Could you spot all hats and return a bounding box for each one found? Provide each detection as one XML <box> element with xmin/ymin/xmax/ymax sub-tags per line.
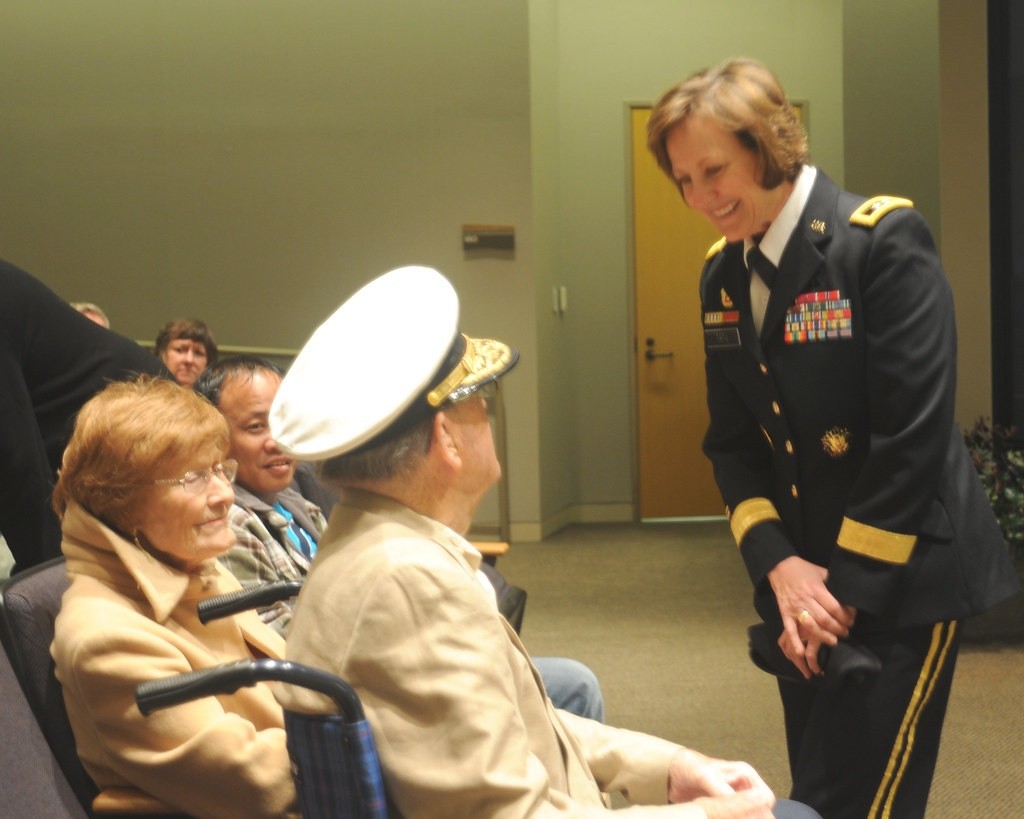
<box><xmin>267</xmin><ymin>265</ymin><xmax>518</xmax><ymax>462</ymax></box>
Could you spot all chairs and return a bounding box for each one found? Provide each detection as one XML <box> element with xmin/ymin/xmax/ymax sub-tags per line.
<box><xmin>2</xmin><ymin>552</ymin><xmax>100</xmax><ymax>808</ymax></box>
<box><xmin>0</xmin><ymin>640</ymin><xmax>92</xmax><ymax>819</ymax></box>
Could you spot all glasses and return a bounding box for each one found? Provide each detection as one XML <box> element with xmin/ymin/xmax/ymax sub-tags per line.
<box><xmin>155</xmin><ymin>458</ymin><xmax>238</xmax><ymax>496</ymax></box>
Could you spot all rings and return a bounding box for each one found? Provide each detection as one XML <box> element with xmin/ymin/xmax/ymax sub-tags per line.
<box><xmin>797</xmin><ymin>609</ymin><xmax>809</xmax><ymax>624</ymax></box>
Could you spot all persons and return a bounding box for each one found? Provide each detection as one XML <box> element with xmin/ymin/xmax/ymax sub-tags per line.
<box><xmin>155</xmin><ymin>319</ymin><xmax>219</xmax><ymax>389</ymax></box>
<box><xmin>50</xmin><ymin>376</ymin><xmax>288</xmax><ymax>819</ymax></box>
<box><xmin>0</xmin><ymin>258</ymin><xmax>178</xmax><ymax>576</ymax></box>
<box><xmin>647</xmin><ymin>60</ymin><xmax>1024</xmax><ymax>819</ymax></box>
<box><xmin>193</xmin><ymin>356</ymin><xmax>604</xmax><ymax>722</ymax></box>
<box><xmin>267</xmin><ymin>264</ymin><xmax>822</xmax><ymax>819</ymax></box>
